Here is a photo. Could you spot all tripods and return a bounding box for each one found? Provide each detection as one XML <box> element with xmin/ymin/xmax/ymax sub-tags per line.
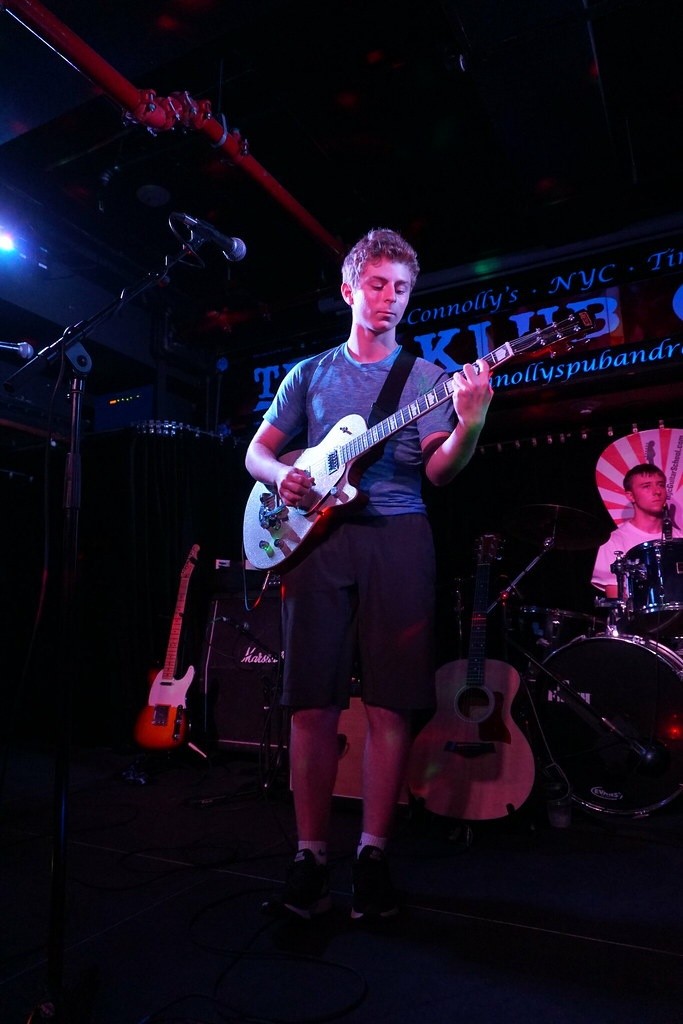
<box><xmin>190</xmin><ymin>624</ymin><xmax>289</xmax><ymax>844</ymax></box>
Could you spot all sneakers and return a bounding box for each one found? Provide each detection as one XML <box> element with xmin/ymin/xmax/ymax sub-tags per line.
<box><xmin>349</xmin><ymin>844</ymin><xmax>397</xmax><ymax>921</ymax></box>
<box><xmin>259</xmin><ymin>847</ymin><xmax>340</xmax><ymax>919</ymax></box>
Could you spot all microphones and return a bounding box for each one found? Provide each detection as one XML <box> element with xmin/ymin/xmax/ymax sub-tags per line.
<box><xmin>662</xmin><ymin>503</ymin><xmax>673</xmax><ymax>541</ymax></box>
<box><xmin>175</xmin><ymin>213</ymin><xmax>246</xmax><ymax>261</ymax></box>
<box><xmin>220</xmin><ymin>615</ymin><xmax>249</xmax><ymax>633</ymax></box>
<box><xmin>640</xmin><ymin>747</ymin><xmax>660</xmax><ymax>767</ymax></box>
<box><xmin>0</xmin><ymin>341</ymin><xmax>34</xmax><ymax>359</ymax></box>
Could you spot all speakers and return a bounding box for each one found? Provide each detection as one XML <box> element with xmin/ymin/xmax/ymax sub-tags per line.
<box><xmin>203</xmin><ymin>588</ymin><xmax>292</xmax><ymax>750</ymax></box>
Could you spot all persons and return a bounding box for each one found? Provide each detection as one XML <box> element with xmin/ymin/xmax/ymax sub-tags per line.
<box><xmin>246</xmin><ymin>230</ymin><xmax>496</xmax><ymax>920</ymax></box>
<box><xmin>590</xmin><ymin>463</ymin><xmax>683</xmax><ymax>602</ymax></box>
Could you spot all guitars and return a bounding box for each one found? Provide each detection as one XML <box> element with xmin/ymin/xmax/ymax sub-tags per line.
<box><xmin>405</xmin><ymin>533</ymin><xmax>536</xmax><ymax>828</ymax></box>
<box><xmin>133</xmin><ymin>543</ymin><xmax>201</xmax><ymax>753</ymax></box>
<box><xmin>243</xmin><ymin>309</ymin><xmax>594</xmax><ymax>576</ymax></box>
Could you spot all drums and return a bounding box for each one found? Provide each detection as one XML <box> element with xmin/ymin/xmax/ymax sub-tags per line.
<box><xmin>529</xmin><ymin>630</ymin><xmax>683</xmax><ymax>820</ymax></box>
<box><xmin>610</xmin><ymin>537</ymin><xmax>683</xmax><ymax>633</ymax></box>
<box><xmin>516</xmin><ymin>604</ymin><xmax>609</xmax><ymax>653</ymax></box>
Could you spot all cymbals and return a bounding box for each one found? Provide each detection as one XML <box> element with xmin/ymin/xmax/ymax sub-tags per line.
<box><xmin>499</xmin><ymin>503</ymin><xmax>610</xmax><ymax>552</ymax></box>
